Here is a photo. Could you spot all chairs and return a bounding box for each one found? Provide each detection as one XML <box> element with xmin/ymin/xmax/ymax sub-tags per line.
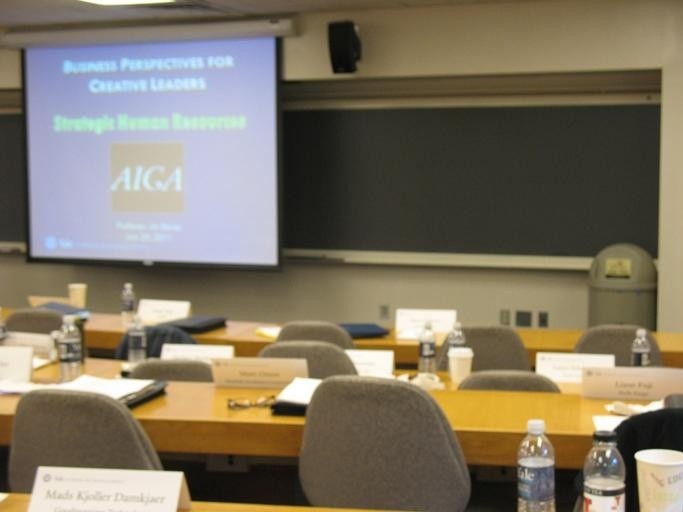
<box><xmin>126</xmin><ymin>358</ymin><xmax>214</xmax><ymax>382</ymax></box>
<box><xmin>256</xmin><ymin>339</ymin><xmax>360</xmax><ymax>374</ymax></box>
<box><xmin>570</xmin><ymin>323</ymin><xmax>665</xmax><ymax>367</ymax></box>
<box><xmin>5</xmin><ymin>389</ymin><xmax>164</xmax><ymax>493</ymax></box>
<box><xmin>4</xmin><ymin>307</ymin><xmax>65</xmax><ymax>334</ymax></box>
<box><xmin>456</xmin><ymin>369</ymin><xmax>562</xmax><ymax>391</ymax></box>
<box><xmin>434</xmin><ymin>326</ymin><xmax>534</xmax><ymax>368</ymax></box>
<box><xmin>297</xmin><ymin>374</ymin><xmax>472</xmax><ymax>510</ymax></box>
<box><xmin>274</xmin><ymin>320</ymin><xmax>355</xmax><ymax>349</ymax></box>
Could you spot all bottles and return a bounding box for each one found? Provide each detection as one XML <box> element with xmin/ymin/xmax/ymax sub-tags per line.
<box><xmin>584</xmin><ymin>430</ymin><xmax>626</xmax><ymax>511</ymax></box>
<box><xmin>448</xmin><ymin>321</ymin><xmax>465</xmax><ymax>348</ymax></box>
<box><xmin>58</xmin><ymin>315</ymin><xmax>81</xmax><ymax>381</ymax></box>
<box><xmin>417</xmin><ymin>323</ymin><xmax>435</xmax><ymax>373</ymax></box>
<box><xmin>122</xmin><ymin>283</ymin><xmax>135</xmax><ymax>326</ymax></box>
<box><xmin>127</xmin><ymin>315</ymin><xmax>147</xmax><ymax>363</ymax></box>
<box><xmin>630</xmin><ymin>329</ymin><xmax>650</xmax><ymax>366</ymax></box>
<box><xmin>516</xmin><ymin>419</ymin><xmax>555</xmax><ymax>511</ymax></box>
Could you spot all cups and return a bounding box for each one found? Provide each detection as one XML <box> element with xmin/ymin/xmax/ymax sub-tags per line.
<box><xmin>66</xmin><ymin>282</ymin><xmax>87</xmax><ymax>309</ymax></box>
<box><xmin>445</xmin><ymin>345</ymin><xmax>473</xmax><ymax>385</ymax></box>
<box><xmin>631</xmin><ymin>448</ymin><xmax>682</xmax><ymax>512</ymax></box>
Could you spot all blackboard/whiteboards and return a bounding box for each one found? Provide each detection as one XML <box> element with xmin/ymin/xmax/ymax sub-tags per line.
<box><xmin>0</xmin><ymin>66</ymin><xmax>662</xmax><ymax>273</ymax></box>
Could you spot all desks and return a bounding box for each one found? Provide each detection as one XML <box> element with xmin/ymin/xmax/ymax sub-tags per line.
<box><xmin>0</xmin><ymin>357</ymin><xmax>683</xmax><ymax>483</ymax></box>
<box><xmin>0</xmin><ymin>306</ymin><xmax>683</xmax><ymax>367</ymax></box>
<box><xmin>0</xmin><ymin>492</ymin><xmax>379</xmax><ymax>511</ymax></box>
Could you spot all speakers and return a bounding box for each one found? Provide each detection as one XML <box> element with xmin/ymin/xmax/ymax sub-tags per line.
<box><xmin>326</xmin><ymin>19</ymin><xmax>361</xmax><ymax>75</ymax></box>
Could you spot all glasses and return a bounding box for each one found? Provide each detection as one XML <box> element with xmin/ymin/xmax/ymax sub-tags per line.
<box><xmin>226</xmin><ymin>393</ymin><xmax>274</xmax><ymax>409</ymax></box>
